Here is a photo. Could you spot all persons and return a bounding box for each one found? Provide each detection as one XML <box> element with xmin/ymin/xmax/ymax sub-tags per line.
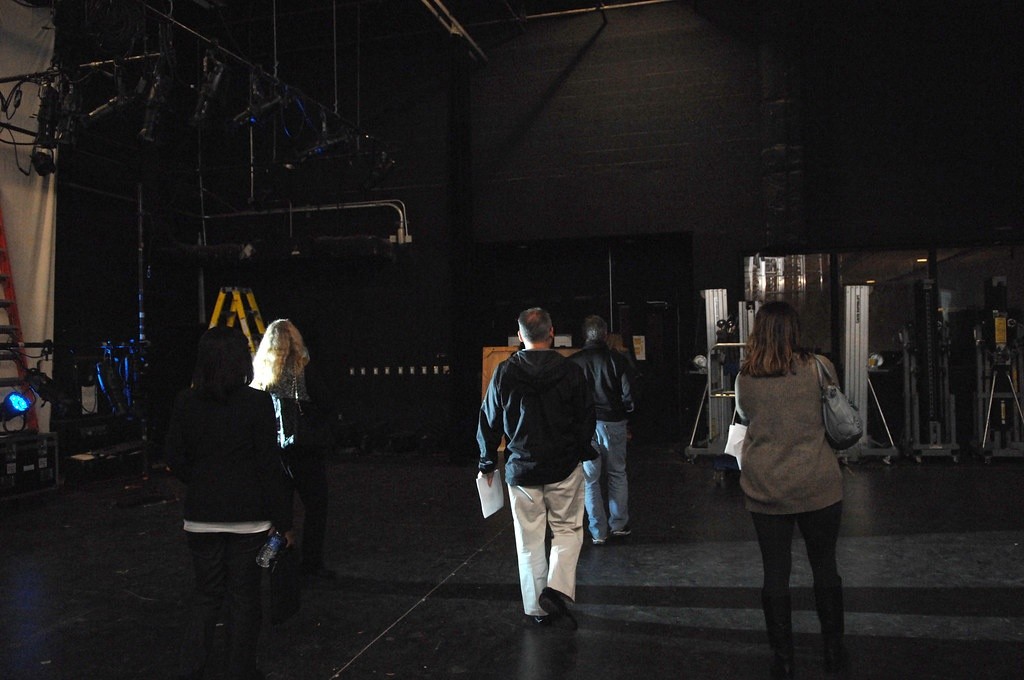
<box><xmin>735</xmin><ymin>301</ymin><xmax>846</xmax><ymax>679</ymax></box>
<box><xmin>165</xmin><ymin>326</ymin><xmax>289</xmax><ymax>680</ymax></box>
<box><xmin>248</xmin><ymin>318</ymin><xmax>337</xmax><ymax>581</ymax></box>
<box><xmin>564</xmin><ymin>312</ymin><xmax>634</xmax><ymax>550</ymax></box>
<box><xmin>477</xmin><ymin>307</ymin><xmax>599</xmax><ymax>630</ymax></box>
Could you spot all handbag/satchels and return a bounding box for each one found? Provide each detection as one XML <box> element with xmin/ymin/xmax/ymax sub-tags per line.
<box><xmin>813</xmin><ymin>354</ymin><xmax>864</xmax><ymax>450</ymax></box>
<box><xmin>275</xmin><ymin>365</ymin><xmax>334</xmax><ymax>453</ymax></box>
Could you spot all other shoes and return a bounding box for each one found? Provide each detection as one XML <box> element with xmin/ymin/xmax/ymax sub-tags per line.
<box><xmin>613</xmin><ymin>525</ymin><xmax>631</xmax><ymax>536</ymax></box>
<box><xmin>286</xmin><ymin>541</ymin><xmax>297</xmax><ymax>551</ymax></box>
<box><xmin>539</xmin><ymin>587</ymin><xmax>578</xmax><ymax>631</ymax></box>
<box><xmin>769</xmin><ymin>663</ymin><xmax>795</xmax><ymax>680</ymax></box>
<box><xmin>592</xmin><ymin>536</ymin><xmax>608</xmax><ymax>545</ymax></box>
<box><xmin>826</xmin><ymin>640</ymin><xmax>849</xmax><ymax>672</ymax></box>
<box><xmin>532</xmin><ymin>615</ymin><xmax>552</xmax><ymax>627</ymax></box>
<box><xmin>301</xmin><ymin>563</ymin><xmax>336</xmax><ymax>578</ymax></box>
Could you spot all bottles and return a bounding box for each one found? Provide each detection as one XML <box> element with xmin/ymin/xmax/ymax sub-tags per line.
<box><xmin>256</xmin><ymin>533</ymin><xmax>286</xmax><ymax>567</ymax></box>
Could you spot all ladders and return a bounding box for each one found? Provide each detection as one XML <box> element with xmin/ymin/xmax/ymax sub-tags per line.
<box><xmin>0</xmin><ymin>208</ymin><xmax>40</xmax><ymax>434</ymax></box>
<box><xmin>191</xmin><ymin>287</ymin><xmax>266</xmax><ymax>387</ymax></box>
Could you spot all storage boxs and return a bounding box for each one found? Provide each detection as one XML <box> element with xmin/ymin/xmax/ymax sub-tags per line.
<box><xmin>0</xmin><ymin>433</ymin><xmax>59</xmax><ymax>502</ymax></box>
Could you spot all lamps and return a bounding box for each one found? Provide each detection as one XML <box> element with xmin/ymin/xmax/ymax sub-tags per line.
<box><xmin>0</xmin><ymin>393</ymin><xmax>30</xmax><ymax>434</ymax></box>
<box><xmin>29</xmin><ymin>55</ymin><xmax>399</xmax><ymax>187</ymax></box>
